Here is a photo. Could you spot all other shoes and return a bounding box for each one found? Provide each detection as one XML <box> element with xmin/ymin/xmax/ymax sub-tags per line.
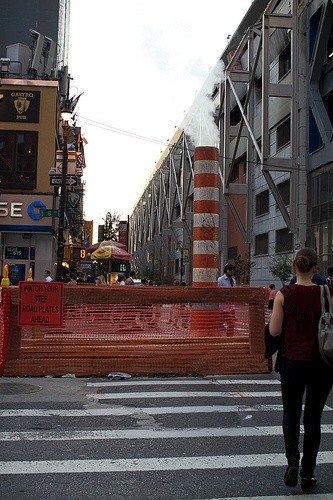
<box><xmin>301</xmin><ymin>477</ymin><xmax>316</xmax><ymax>492</ymax></box>
<box><xmin>284</xmin><ymin>463</ymin><xmax>298</xmax><ymax>487</ymax></box>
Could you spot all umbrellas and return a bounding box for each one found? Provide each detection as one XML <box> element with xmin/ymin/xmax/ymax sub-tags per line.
<box><xmin>90</xmin><ymin>245</ymin><xmax>133</xmax><ymax>273</ymax></box>
<box><xmin>86</xmin><ymin>240</ymin><xmax>127</xmax><ymax>274</ymax></box>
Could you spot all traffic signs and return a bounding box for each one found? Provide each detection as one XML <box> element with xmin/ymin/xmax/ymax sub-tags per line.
<box><xmin>50</xmin><ymin>176</ymin><xmax>77</xmax><ymax>186</ymax></box>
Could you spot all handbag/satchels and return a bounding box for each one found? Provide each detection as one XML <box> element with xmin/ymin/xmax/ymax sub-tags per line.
<box><xmin>317</xmin><ymin>285</ymin><xmax>333</xmax><ymax>365</ymax></box>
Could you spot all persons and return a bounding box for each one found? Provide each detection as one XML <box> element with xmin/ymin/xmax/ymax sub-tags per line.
<box><xmin>265</xmin><ymin>247</ymin><xmax>333</xmax><ymax>491</ymax></box>
<box><xmin>43</xmin><ymin>268</ymin><xmax>191</xmax><ymax>333</ymax></box>
<box><xmin>289</xmin><ymin>260</ymin><xmax>333</xmax><ymax>296</ymax></box>
<box><xmin>8</xmin><ymin>270</ymin><xmax>19</xmax><ymax>286</ymax></box>
<box><xmin>216</xmin><ymin>264</ymin><xmax>237</xmax><ymax>336</ymax></box>
<box><xmin>267</xmin><ymin>284</ymin><xmax>276</xmax><ymax>318</ymax></box>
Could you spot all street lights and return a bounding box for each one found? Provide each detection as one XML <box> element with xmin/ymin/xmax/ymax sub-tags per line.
<box><xmin>55</xmin><ymin>99</ymin><xmax>73</xmax><ymax>281</ymax></box>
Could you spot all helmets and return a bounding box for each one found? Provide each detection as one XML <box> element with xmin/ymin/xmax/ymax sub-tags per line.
<box><xmin>225</xmin><ymin>264</ymin><xmax>233</xmax><ymax>269</ymax></box>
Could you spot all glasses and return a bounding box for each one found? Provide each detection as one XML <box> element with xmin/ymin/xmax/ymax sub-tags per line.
<box><xmin>227</xmin><ymin>268</ymin><xmax>236</xmax><ymax>271</ymax></box>
